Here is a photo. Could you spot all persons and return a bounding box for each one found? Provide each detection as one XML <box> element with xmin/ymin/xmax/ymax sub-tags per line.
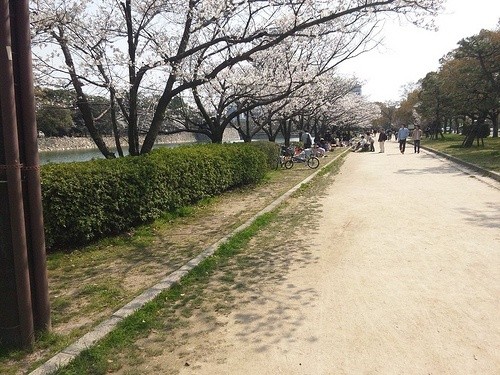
<box><xmin>412</xmin><ymin>125</ymin><xmax>422</xmax><ymax>153</ymax></box>
<box><xmin>278</xmin><ymin>128</ymin><xmax>398</xmax><ymax>160</ymax></box>
<box><xmin>398</xmin><ymin>124</ymin><xmax>410</xmax><ymax>153</ymax></box>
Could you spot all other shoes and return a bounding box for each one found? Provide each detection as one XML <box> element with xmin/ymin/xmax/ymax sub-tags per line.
<box><xmin>379</xmin><ymin>152</ymin><xmax>384</xmax><ymax>153</ymax></box>
<box><xmin>352</xmin><ymin>150</ymin><xmax>354</xmax><ymax>151</ymax></box>
<box><xmin>401</xmin><ymin>151</ymin><xmax>404</xmax><ymax>153</ymax></box>
<box><xmin>415</xmin><ymin>151</ymin><xmax>419</xmax><ymax>153</ymax></box>
<box><xmin>370</xmin><ymin>149</ymin><xmax>374</xmax><ymax>151</ymax></box>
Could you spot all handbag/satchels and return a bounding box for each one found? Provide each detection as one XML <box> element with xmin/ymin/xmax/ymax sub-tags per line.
<box><xmin>307</xmin><ymin>134</ymin><xmax>312</xmax><ymax>145</ymax></box>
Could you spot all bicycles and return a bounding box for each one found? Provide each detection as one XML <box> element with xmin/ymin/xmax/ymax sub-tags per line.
<box><xmin>283</xmin><ymin>142</ymin><xmax>320</xmax><ymax>169</ymax></box>
